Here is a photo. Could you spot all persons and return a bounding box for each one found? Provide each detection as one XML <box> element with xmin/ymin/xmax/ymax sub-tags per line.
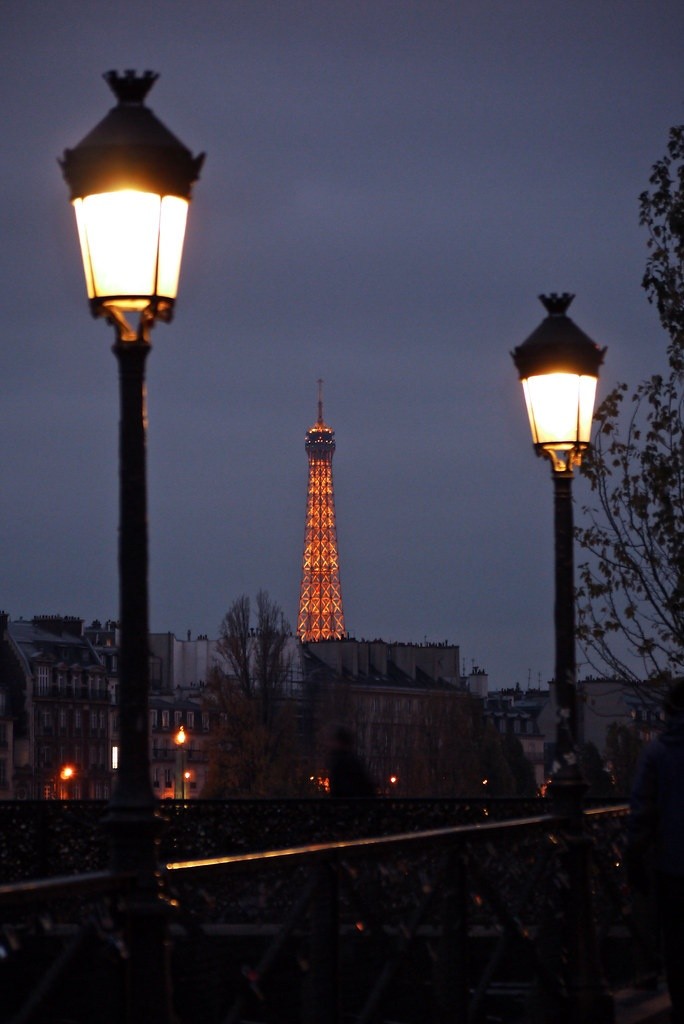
<box><xmin>328</xmin><ymin>725</ymin><xmax>379</xmax><ymax>798</ymax></box>
<box><xmin>629</xmin><ymin>676</ymin><xmax>684</xmax><ymax>1024</ymax></box>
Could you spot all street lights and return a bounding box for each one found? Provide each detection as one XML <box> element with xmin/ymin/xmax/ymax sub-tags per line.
<box><xmin>174</xmin><ymin>726</ymin><xmax>186</xmax><ymax>800</ymax></box>
<box><xmin>509</xmin><ymin>287</ymin><xmax>618</xmax><ymax>807</ymax></box>
<box><xmin>46</xmin><ymin>59</ymin><xmax>214</xmax><ymax>1024</ymax></box>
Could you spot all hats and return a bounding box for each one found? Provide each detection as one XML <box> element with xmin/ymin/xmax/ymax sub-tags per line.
<box><xmin>662</xmin><ymin>679</ymin><xmax>684</xmax><ymax>718</ymax></box>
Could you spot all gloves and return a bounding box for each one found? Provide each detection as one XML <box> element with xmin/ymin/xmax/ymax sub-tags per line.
<box><xmin>623</xmin><ymin>849</ymin><xmax>649</xmax><ymax>898</ymax></box>
